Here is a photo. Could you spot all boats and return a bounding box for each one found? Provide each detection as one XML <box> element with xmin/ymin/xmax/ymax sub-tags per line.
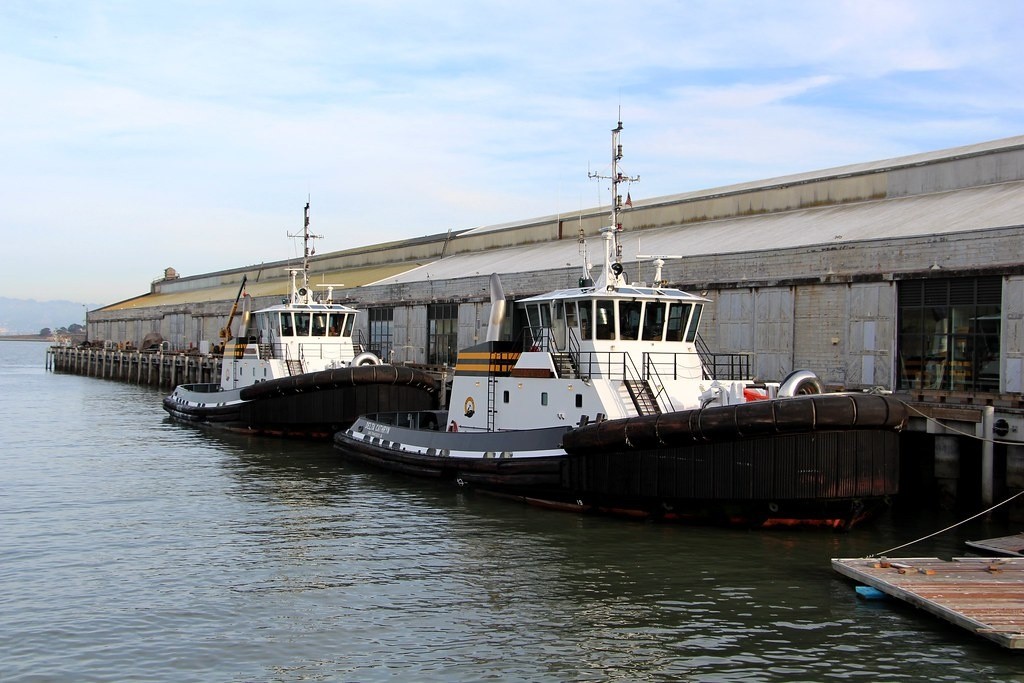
<box><xmin>162</xmin><ymin>193</ymin><xmax>440</xmax><ymax>440</ymax></box>
<box><xmin>333</xmin><ymin>106</ymin><xmax>907</xmax><ymax>537</ymax></box>
